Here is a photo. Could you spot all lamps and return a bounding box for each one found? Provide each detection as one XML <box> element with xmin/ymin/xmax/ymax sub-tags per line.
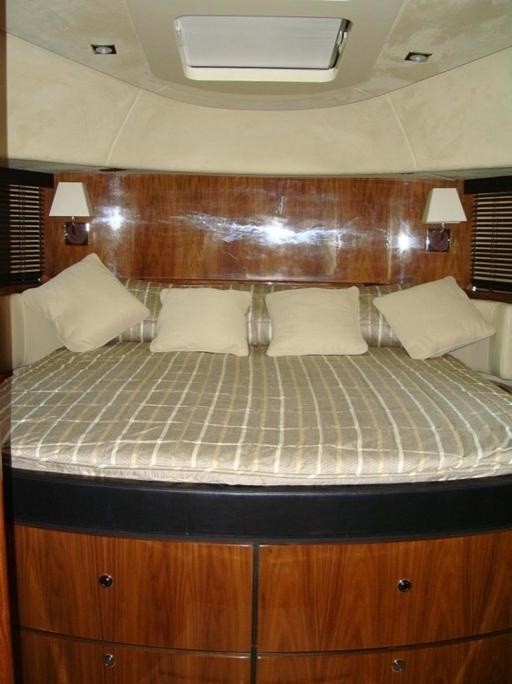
<box><xmin>422</xmin><ymin>186</ymin><xmax>469</xmax><ymax>254</ymax></box>
<box><xmin>48</xmin><ymin>178</ymin><xmax>93</xmax><ymax>246</ymax></box>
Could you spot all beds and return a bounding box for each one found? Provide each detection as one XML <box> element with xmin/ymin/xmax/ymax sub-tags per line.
<box><xmin>0</xmin><ymin>270</ymin><xmax>512</xmax><ymax>682</ymax></box>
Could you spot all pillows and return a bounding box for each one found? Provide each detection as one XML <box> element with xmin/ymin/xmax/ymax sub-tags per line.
<box><xmin>22</xmin><ymin>249</ymin><xmax>149</xmax><ymax>354</ymax></box>
<box><xmin>150</xmin><ymin>289</ymin><xmax>250</xmax><ymax>358</ymax></box>
<box><xmin>372</xmin><ymin>275</ymin><xmax>499</xmax><ymax>360</ymax></box>
<box><xmin>262</xmin><ymin>285</ymin><xmax>369</xmax><ymax>358</ymax></box>
